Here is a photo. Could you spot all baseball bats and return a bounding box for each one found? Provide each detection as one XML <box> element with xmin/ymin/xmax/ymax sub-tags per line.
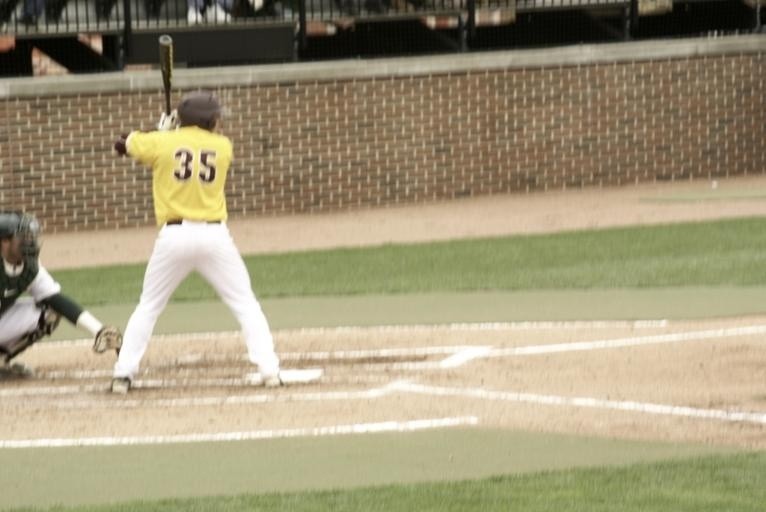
<box><xmin>157</xmin><ymin>35</ymin><xmax>174</xmax><ymax>118</ymax></box>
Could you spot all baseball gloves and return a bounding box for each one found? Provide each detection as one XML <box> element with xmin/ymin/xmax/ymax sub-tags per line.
<box><xmin>91</xmin><ymin>323</ymin><xmax>123</xmax><ymax>353</ymax></box>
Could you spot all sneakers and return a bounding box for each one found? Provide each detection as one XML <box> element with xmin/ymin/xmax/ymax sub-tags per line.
<box><xmin>111</xmin><ymin>377</ymin><xmax>130</xmax><ymax>394</ymax></box>
<box><xmin>0</xmin><ymin>362</ymin><xmax>33</xmax><ymax>378</ymax></box>
<box><xmin>266</xmin><ymin>376</ymin><xmax>284</xmax><ymax>388</ymax></box>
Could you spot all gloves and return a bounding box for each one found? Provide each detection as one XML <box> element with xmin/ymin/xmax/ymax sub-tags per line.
<box><xmin>157</xmin><ymin>108</ymin><xmax>181</xmax><ymax>130</ymax></box>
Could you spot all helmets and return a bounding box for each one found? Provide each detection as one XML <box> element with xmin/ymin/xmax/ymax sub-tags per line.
<box><xmin>178</xmin><ymin>94</ymin><xmax>221</xmax><ymax>120</ymax></box>
<box><xmin>1</xmin><ymin>209</ymin><xmax>39</xmax><ymax>276</ymax></box>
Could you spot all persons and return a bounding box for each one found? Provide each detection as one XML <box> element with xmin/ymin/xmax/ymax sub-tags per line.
<box><xmin>0</xmin><ymin>209</ymin><xmax>123</xmax><ymax>380</ymax></box>
<box><xmin>108</xmin><ymin>89</ymin><xmax>282</xmax><ymax>394</ymax></box>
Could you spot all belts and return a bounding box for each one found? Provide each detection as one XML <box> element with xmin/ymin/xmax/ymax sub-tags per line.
<box><xmin>168</xmin><ymin>220</ymin><xmax>221</xmax><ymax>223</ymax></box>
